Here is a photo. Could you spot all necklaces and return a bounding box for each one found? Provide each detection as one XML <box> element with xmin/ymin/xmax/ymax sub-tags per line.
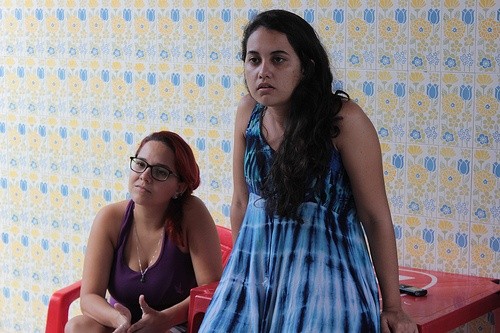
<box><xmin>134</xmin><ymin>224</ymin><xmax>163</xmax><ymax>282</ymax></box>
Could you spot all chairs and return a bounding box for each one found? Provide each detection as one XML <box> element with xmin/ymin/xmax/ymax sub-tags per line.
<box><xmin>45</xmin><ymin>225</ymin><xmax>233</xmax><ymax>333</ymax></box>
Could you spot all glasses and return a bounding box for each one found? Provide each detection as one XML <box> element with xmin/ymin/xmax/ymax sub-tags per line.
<box><xmin>130</xmin><ymin>156</ymin><xmax>180</xmax><ymax>181</ymax></box>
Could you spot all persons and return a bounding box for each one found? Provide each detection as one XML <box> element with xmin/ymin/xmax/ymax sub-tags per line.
<box><xmin>198</xmin><ymin>10</ymin><xmax>418</xmax><ymax>333</ymax></box>
<box><xmin>64</xmin><ymin>131</ymin><xmax>222</xmax><ymax>333</ymax></box>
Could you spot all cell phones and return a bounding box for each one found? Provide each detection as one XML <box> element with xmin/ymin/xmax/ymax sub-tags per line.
<box><xmin>400</xmin><ymin>284</ymin><xmax>428</xmax><ymax>296</ymax></box>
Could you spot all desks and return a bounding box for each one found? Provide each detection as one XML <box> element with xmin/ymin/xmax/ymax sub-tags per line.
<box><xmin>190</xmin><ymin>258</ymin><xmax>500</xmax><ymax>333</ymax></box>
